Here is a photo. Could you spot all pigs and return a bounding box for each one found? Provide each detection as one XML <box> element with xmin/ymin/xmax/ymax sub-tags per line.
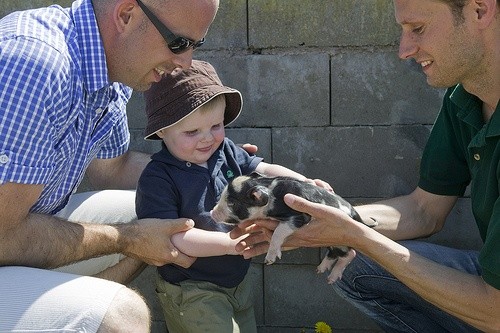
<box><xmin>210</xmin><ymin>171</ymin><xmax>378</xmax><ymax>285</ymax></box>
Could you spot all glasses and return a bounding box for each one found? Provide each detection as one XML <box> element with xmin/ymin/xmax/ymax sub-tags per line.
<box><xmin>135</xmin><ymin>0</ymin><xmax>206</xmax><ymax>54</ymax></box>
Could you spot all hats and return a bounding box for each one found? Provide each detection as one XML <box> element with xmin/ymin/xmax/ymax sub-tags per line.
<box><xmin>143</xmin><ymin>59</ymin><xmax>243</xmax><ymax>140</ymax></box>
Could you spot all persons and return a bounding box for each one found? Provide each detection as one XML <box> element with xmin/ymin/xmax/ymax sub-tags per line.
<box><xmin>0</xmin><ymin>0</ymin><xmax>255</xmax><ymax>333</ymax></box>
<box><xmin>228</xmin><ymin>0</ymin><xmax>500</xmax><ymax>333</ymax></box>
<box><xmin>135</xmin><ymin>61</ymin><xmax>338</xmax><ymax>333</ymax></box>
